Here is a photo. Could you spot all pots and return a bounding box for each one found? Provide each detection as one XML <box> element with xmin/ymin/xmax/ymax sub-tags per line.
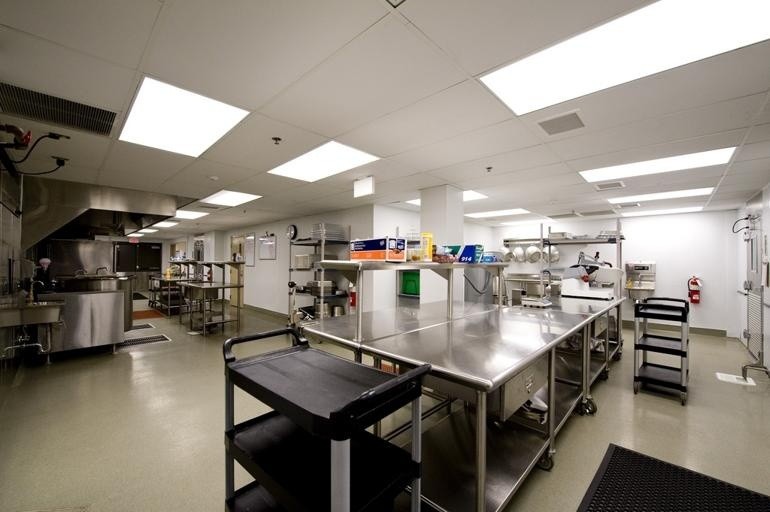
<box><xmin>498</xmin><ymin>244</ymin><xmax>560</xmax><ymax>265</ymax></box>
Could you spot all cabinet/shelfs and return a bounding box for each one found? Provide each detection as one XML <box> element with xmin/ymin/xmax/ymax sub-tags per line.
<box><xmin>633</xmin><ymin>296</ymin><xmax>689</xmax><ymax>406</ymax></box>
<box><xmin>148</xmin><ymin>260</ymin><xmax>245</xmax><ymax>337</ymax></box>
<box><xmin>287</xmin><ymin>237</ymin><xmax>357</xmax><ymax>345</ymax></box>
<box><xmin>503</xmin><ymin>218</ymin><xmax>623</xmax><ymax>306</ymax></box>
<box><xmin>222</xmin><ymin>327</ymin><xmax>430</xmax><ymax>512</ymax></box>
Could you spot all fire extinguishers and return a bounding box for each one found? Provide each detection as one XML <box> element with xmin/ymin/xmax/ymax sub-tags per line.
<box><xmin>688</xmin><ymin>276</ymin><xmax>703</xmax><ymax>303</ymax></box>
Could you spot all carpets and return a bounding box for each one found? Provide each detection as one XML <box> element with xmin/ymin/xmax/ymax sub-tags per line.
<box><xmin>575</xmin><ymin>441</ymin><xmax>769</xmax><ymax>511</ymax></box>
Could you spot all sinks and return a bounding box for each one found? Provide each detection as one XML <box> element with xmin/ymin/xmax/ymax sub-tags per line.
<box><xmin>0</xmin><ymin>301</ymin><xmax>65</xmax><ymax>327</ymax></box>
<box><xmin>55</xmin><ymin>274</ymin><xmax>137</xmax><ymax>332</ymax></box>
<box><xmin>624</xmin><ymin>280</ymin><xmax>656</xmax><ymax>300</ymax></box>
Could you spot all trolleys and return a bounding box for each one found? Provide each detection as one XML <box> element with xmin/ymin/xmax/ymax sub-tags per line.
<box><xmin>222</xmin><ymin>325</ymin><xmax>434</xmax><ymax>512</ymax></box>
<box><xmin>633</xmin><ymin>296</ymin><xmax>690</xmax><ymax>407</ymax></box>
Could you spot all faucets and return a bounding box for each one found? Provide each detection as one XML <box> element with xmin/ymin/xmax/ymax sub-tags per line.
<box><xmin>639</xmin><ymin>275</ymin><xmax>642</xmax><ymax>286</ymax></box>
<box><xmin>95</xmin><ymin>267</ymin><xmax>108</xmax><ymax>274</ymax></box>
<box><xmin>74</xmin><ymin>269</ymin><xmax>87</xmax><ymax>275</ymax></box>
<box><xmin>28</xmin><ymin>281</ymin><xmax>45</xmax><ymax>302</ymax></box>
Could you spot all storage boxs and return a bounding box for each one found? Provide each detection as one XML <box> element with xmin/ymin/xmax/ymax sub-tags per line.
<box><xmin>348</xmin><ymin>236</ymin><xmax>407</xmax><ymax>262</ymax></box>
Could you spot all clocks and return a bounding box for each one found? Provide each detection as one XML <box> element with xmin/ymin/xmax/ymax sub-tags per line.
<box><xmin>285</xmin><ymin>224</ymin><xmax>297</xmax><ymax>240</ymax></box>
